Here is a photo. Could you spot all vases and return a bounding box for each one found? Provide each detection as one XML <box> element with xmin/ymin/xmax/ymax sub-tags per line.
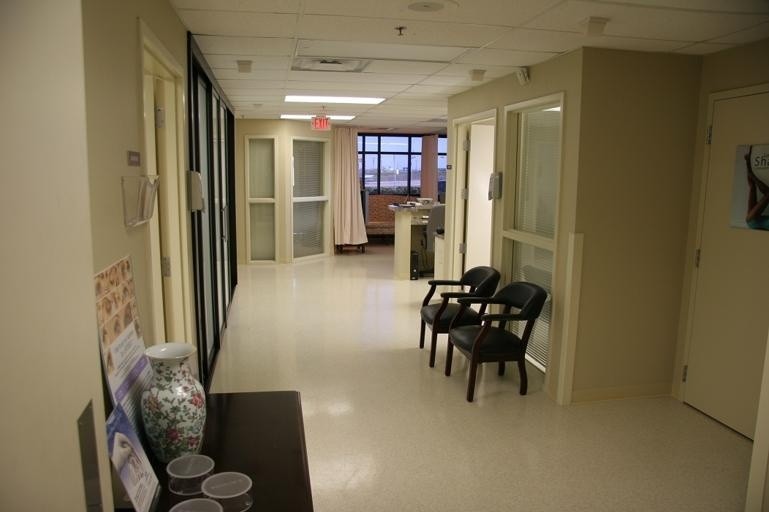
<box><xmin>140</xmin><ymin>341</ymin><xmax>208</xmax><ymax>464</ymax></box>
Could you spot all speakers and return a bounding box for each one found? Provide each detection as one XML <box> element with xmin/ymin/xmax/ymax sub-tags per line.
<box><xmin>516</xmin><ymin>67</ymin><xmax>531</xmax><ymax>86</ymax></box>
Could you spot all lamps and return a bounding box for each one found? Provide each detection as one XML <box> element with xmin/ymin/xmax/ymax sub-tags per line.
<box><xmin>309</xmin><ymin>105</ymin><xmax>332</xmax><ymax>133</ymax></box>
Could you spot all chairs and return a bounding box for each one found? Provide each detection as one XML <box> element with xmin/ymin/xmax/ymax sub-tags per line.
<box><xmin>420</xmin><ymin>205</ymin><xmax>444</xmax><ymax>276</ymax></box>
<box><xmin>419</xmin><ymin>266</ymin><xmax>548</xmax><ymax>402</ymax></box>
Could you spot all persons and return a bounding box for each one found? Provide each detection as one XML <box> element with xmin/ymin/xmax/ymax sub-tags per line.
<box><xmin>111</xmin><ymin>428</ymin><xmax>135</xmax><ymax>477</ymax></box>
<box><xmin>743</xmin><ymin>145</ymin><xmax>769</xmax><ymax>232</ymax></box>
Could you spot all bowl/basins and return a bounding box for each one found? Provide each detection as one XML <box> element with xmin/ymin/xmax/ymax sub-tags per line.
<box><xmin>416</xmin><ymin>198</ymin><xmax>433</xmax><ymax>205</ymax></box>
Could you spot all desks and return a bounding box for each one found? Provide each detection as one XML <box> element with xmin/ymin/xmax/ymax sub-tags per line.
<box><xmin>114</xmin><ymin>390</ymin><xmax>315</xmax><ymax>512</ymax></box>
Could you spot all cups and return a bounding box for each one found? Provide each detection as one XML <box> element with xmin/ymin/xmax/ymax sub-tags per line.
<box><xmin>436</xmin><ymin>223</ymin><xmax>444</xmax><ymax>234</ymax></box>
